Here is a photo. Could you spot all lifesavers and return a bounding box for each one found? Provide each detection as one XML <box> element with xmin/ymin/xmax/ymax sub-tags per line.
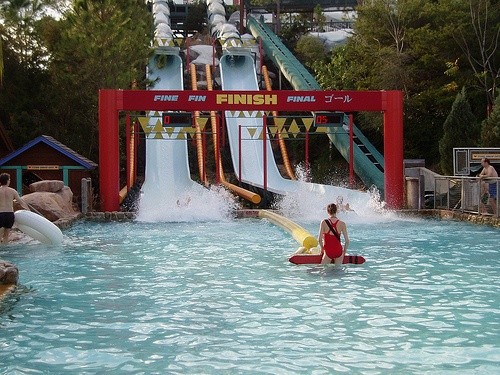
<box><xmin>14</xmin><ymin>210</ymin><xmax>62</xmax><ymax>243</ymax></box>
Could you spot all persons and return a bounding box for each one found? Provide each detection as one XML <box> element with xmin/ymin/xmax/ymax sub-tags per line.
<box><xmin>177</xmin><ymin>196</ymin><xmax>191</xmax><ymax>208</ymax></box>
<box><xmin>318</xmin><ymin>203</ymin><xmax>349</xmax><ymax>265</ymax></box>
<box><xmin>324</xmin><ymin>195</ymin><xmax>354</xmax><ymax>212</ymax></box>
<box><xmin>0</xmin><ymin>173</ymin><xmax>29</xmax><ymax>243</ymax></box>
<box><xmin>476</xmin><ymin>157</ymin><xmax>498</xmax><ymax>217</ymax></box>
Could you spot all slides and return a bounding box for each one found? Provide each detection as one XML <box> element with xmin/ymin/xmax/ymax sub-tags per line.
<box><xmin>219</xmin><ymin>55</ymin><xmax>370</xmax><ymax>218</ymax></box>
<box><xmin>139</xmin><ymin>54</ymin><xmax>208</xmax><ymax>213</ymax></box>
